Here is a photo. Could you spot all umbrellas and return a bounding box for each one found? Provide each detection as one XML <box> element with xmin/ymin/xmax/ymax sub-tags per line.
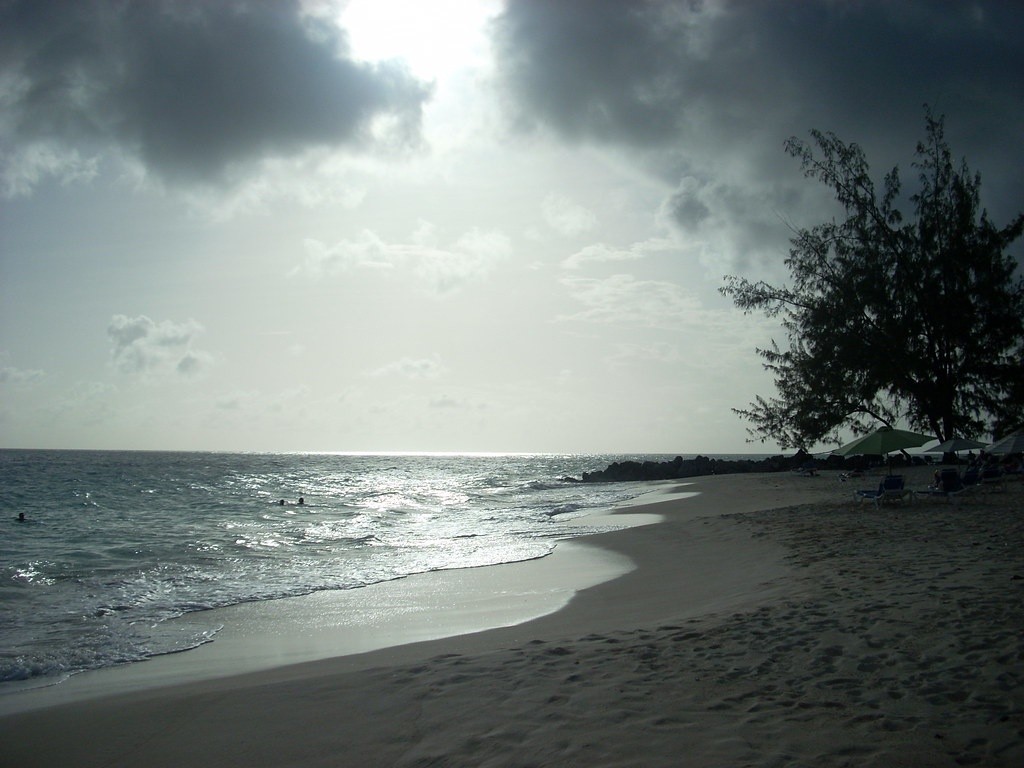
<box><xmin>986</xmin><ymin>429</ymin><xmax>1023</xmax><ymax>462</ymax></box>
<box><xmin>828</xmin><ymin>427</ymin><xmax>937</xmax><ymax>477</ymax></box>
<box><xmin>926</xmin><ymin>435</ymin><xmax>990</xmax><ymax>468</ymax></box>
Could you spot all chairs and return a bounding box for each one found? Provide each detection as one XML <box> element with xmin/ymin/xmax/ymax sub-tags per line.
<box><xmin>852</xmin><ymin>468</ymin><xmax>1008</xmax><ymax>511</ymax></box>
<box><xmin>790</xmin><ymin>468</ymin><xmax>817</xmax><ymax>478</ymax></box>
